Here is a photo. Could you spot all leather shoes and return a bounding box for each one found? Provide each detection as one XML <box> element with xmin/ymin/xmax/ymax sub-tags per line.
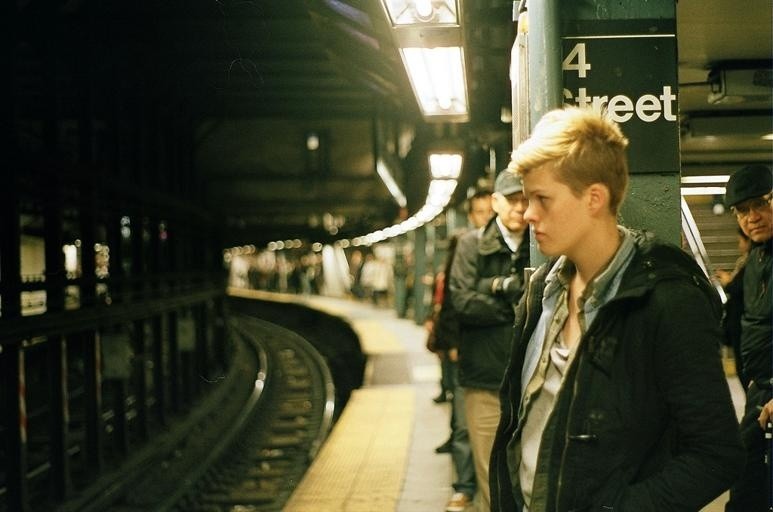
<box><xmin>432</xmin><ymin>390</ymin><xmax>446</xmax><ymax>402</ymax></box>
<box><xmin>437</xmin><ymin>434</ymin><xmax>454</xmax><ymax>454</ymax></box>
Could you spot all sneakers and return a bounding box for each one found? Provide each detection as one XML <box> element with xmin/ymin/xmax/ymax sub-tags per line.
<box><xmin>445</xmin><ymin>492</ymin><xmax>473</xmax><ymax>512</ymax></box>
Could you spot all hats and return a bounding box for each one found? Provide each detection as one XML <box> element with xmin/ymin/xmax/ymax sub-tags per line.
<box><xmin>494</xmin><ymin>168</ymin><xmax>524</xmax><ymax>197</ymax></box>
<box><xmin>725</xmin><ymin>164</ymin><xmax>772</xmax><ymax>209</ymax></box>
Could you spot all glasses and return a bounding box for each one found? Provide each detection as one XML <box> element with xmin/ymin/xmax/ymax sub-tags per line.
<box><xmin>735</xmin><ymin>193</ymin><xmax>773</xmax><ymax>214</ymax></box>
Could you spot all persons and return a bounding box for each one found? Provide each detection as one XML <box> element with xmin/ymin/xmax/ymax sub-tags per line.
<box><xmin>488</xmin><ymin>108</ymin><xmax>746</xmax><ymax>512</ymax></box>
<box><xmin>432</xmin><ymin>168</ymin><xmax>530</xmax><ymax>512</ymax></box>
<box><xmin>716</xmin><ymin>165</ymin><xmax>773</xmax><ymax>512</ymax></box>
